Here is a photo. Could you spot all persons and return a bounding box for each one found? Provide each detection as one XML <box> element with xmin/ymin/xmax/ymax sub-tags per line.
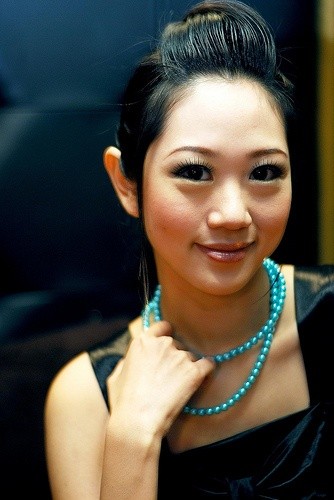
<box><xmin>44</xmin><ymin>0</ymin><xmax>334</xmax><ymax>500</ymax></box>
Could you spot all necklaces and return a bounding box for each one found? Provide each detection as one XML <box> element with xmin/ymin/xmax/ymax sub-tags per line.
<box><xmin>129</xmin><ymin>260</ymin><xmax>286</xmax><ymax>415</ymax></box>
